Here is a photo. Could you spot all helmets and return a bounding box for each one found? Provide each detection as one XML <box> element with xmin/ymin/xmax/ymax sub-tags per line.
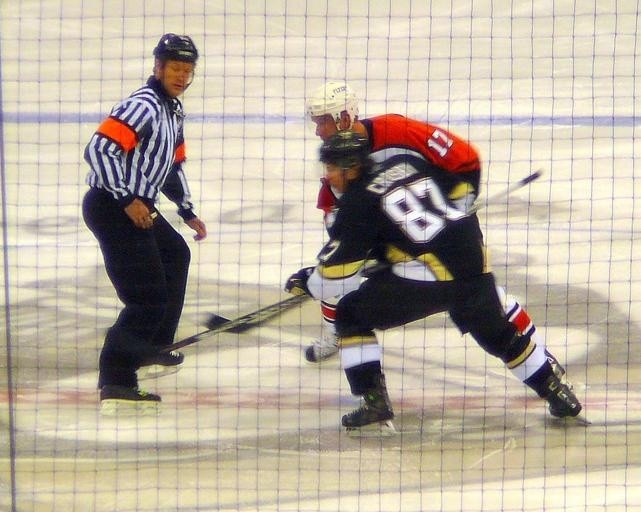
<box><xmin>152</xmin><ymin>32</ymin><xmax>197</xmax><ymax>62</ymax></box>
<box><xmin>305</xmin><ymin>82</ymin><xmax>357</xmax><ymax>117</ymax></box>
<box><xmin>318</xmin><ymin>131</ymin><xmax>370</xmax><ymax>165</ymax></box>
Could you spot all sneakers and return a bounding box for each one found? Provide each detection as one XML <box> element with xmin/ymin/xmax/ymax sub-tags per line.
<box><xmin>137</xmin><ymin>350</ymin><xmax>184</xmax><ymax>366</ymax></box>
<box><xmin>100</xmin><ymin>384</ymin><xmax>160</xmax><ymax>405</ymax></box>
<box><xmin>304</xmin><ymin>337</ymin><xmax>339</xmax><ymax>362</ymax></box>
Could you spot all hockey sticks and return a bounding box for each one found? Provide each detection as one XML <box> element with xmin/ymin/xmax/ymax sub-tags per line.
<box><xmin>135</xmin><ymin>291</ymin><xmax>311</xmax><ymax>361</ymax></box>
<box><xmin>200</xmin><ymin>168</ymin><xmax>539</xmax><ymax>334</ymax></box>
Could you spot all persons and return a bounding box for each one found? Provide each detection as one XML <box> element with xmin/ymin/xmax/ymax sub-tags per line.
<box><xmin>282</xmin><ymin>129</ymin><xmax>590</xmax><ymax>437</ymax></box>
<box><xmin>304</xmin><ymin>79</ymin><xmax>482</xmax><ymax>366</ymax></box>
<box><xmin>79</xmin><ymin>32</ymin><xmax>207</xmax><ymax>416</ymax></box>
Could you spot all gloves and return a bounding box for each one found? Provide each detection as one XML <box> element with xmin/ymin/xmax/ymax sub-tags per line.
<box><xmin>283</xmin><ymin>267</ymin><xmax>315</xmax><ymax>298</ymax></box>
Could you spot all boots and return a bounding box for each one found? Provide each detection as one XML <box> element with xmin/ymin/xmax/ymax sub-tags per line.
<box><xmin>539</xmin><ymin>374</ymin><xmax>581</xmax><ymax>417</ymax></box>
<box><xmin>341</xmin><ymin>375</ymin><xmax>394</xmax><ymax>428</ymax></box>
